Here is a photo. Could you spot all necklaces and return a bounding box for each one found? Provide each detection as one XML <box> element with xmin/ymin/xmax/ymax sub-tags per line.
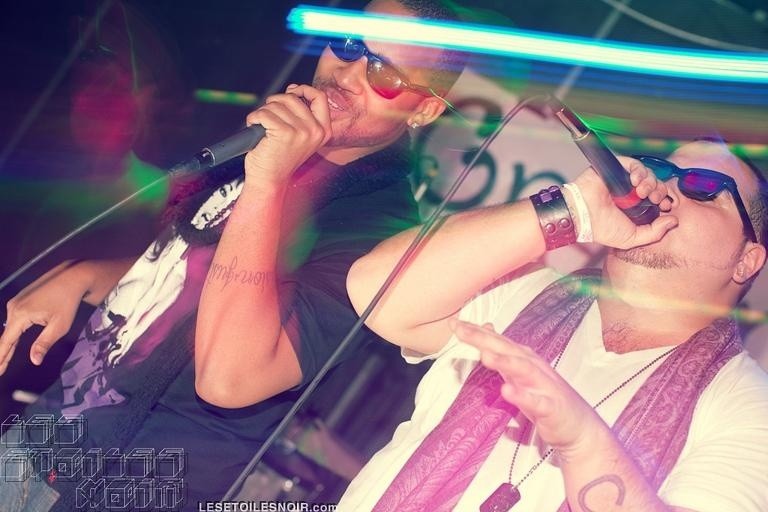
<box><xmin>479</xmin><ymin>295</ymin><xmax>687</xmax><ymax>512</ymax></box>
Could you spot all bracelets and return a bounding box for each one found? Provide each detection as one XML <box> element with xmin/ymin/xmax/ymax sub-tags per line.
<box><xmin>529</xmin><ymin>185</ymin><xmax>576</xmax><ymax>251</ymax></box>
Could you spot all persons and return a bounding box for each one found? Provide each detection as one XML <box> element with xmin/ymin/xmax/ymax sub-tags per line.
<box><xmin>346</xmin><ymin>137</ymin><xmax>768</xmax><ymax>512</ymax></box>
<box><xmin>0</xmin><ymin>1</ymin><xmax>470</xmax><ymax>511</ymax></box>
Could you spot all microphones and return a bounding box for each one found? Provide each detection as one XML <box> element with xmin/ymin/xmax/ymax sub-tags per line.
<box><xmin>168</xmin><ymin>96</ymin><xmax>310</xmax><ymax>178</ymax></box>
<box><xmin>542</xmin><ymin>90</ymin><xmax>660</xmax><ymax>225</ymax></box>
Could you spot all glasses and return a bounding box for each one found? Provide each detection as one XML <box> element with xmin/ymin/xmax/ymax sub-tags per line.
<box><xmin>328</xmin><ymin>34</ymin><xmax>440</xmax><ymax>99</ymax></box>
<box><xmin>631</xmin><ymin>154</ymin><xmax>758</xmax><ymax>243</ymax></box>
